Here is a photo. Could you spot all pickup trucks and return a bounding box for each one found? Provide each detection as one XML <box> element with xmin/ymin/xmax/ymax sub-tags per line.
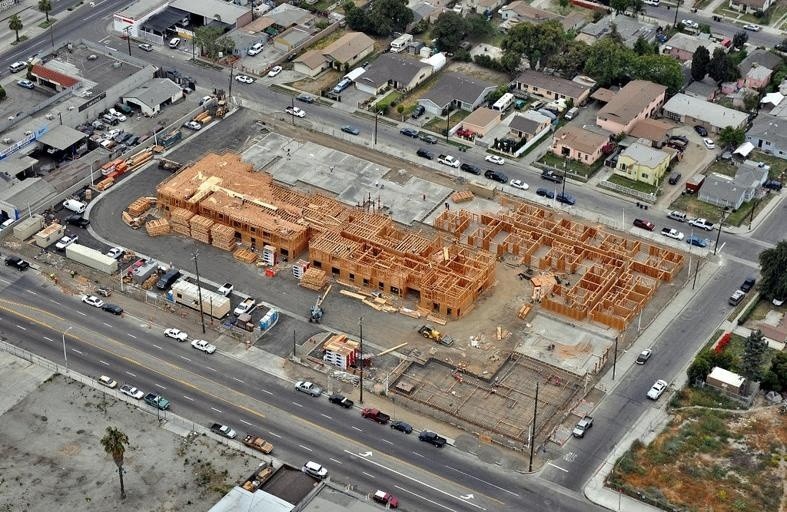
<box><xmin>572</xmin><ymin>415</ymin><xmax>593</xmax><ymax>439</ymax></box>
<box><xmin>292</xmin><ymin>380</ymin><xmax>446</xmax><ymax>448</ymax></box>
<box><xmin>64</xmin><ymin>215</ymin><xmax>89</xmax><ymax>229</ymax></box>
<box><xmin>215</xmin><ymin>282</ymin><xmax>233</xmax><ymax>298</ymax></box>
<box><xmin>143</xmin><ymin>392</ymin><xmax>170</xmax><ymax>410</ymax></box>
<box><xmin>243</xmin><ymin>434</ymin><xmax>273</xmax><ymax>454</ymax></box>
<box><xmin>233</xmin><ymin>297</ymin><xmax>255</xmax><ymax>317</ymax></box>
<box><xmin>4</xmin><ymin>255</ymin><xmax>30</xmax><ymax>272</ymax></box>
<box><xmin>119</xmin><ymin>383</ymin><xmax>144</xmax><ymax>400</ymax></box>
<box><xmin>210</xmin><ymin>422</ymin><xmax>236</xmax><ymax>439</ymax></box>
<box><xmin>54</xmin><ymin>233</ymin><xmax>77</xmax><ymax>249</ymax></box>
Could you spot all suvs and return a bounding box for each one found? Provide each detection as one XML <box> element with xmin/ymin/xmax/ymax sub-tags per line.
<box><xmin>727</xmin><ymin>289</ymin><xmax>745</xmax><ymax>306</ymax></box>
<box><xmin>301</xmin><ymin>461</ymin><xmax>327</xmax><ymax>478</ymax></box>
<box><xmin>740</xmin><ymin>278</ymin><xmax>754</xmax><ymax>293</ymax></box>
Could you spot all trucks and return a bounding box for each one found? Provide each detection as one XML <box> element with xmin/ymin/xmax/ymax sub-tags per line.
<box><xmin>373</xmin><ymin>489</ymin><xmax>398</xmax><ymax>507</ymax></box>
<box><xmin>156</xmin><ymin>269</ymin><xmax>179</xmax><ymax>290</ymax></box>
<box><xmin>407</xmin><ymin>41</ymin><xmax>425</xmax><ymax>54</ymax></box>
<box><xmin>389</xmin><ymin>33</ymin><xmax>413</xmax><ymax>53</ymax></box>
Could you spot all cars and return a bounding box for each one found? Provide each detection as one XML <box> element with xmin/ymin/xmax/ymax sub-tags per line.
<box><xmin>267</xmin><ymin>66</ymin><xmax>282</xmax><ymax>78</ymax></box>
<box><xmin>632</xmin><ymin>208</ymin><xmax>713</xmax><ymax>248</ymax></box>
<box><xmin>295</xmin><ymin>94</ymin><xmax>313</xmax><ymax>104</ymax></box>
<box><xmin>138</xmin><ymin>43</ymin><xmax>153</xmax><ymax>52</ymax></box>
<box><xmin>105</xmin><ymin>246</ymin><xmax>124</xmax><ymax>259</ymax></box>
<box><xmin>284</xmin><ymin>105</ymin><xmax>306</xmax><ymax>118</ymax></box>
<box><xmin>16</xmin><ymin>79</ymin><xmax>34</xmax><ymax>90</ymax></box>
<box><xmin>76</xmin><ymin>103</ymin><xmax>163</xmax><ymax>152</ymax></box>
<box><xmin>703</xmin><ymin>137</ymin><xmax>715</xmax><ymax>150</ymax></box>
<box><xmin>340</xmin><ymin>124</ymin><xmax>359</xmax><ymax>136</ymax></box>
<box><xmin>190</xmin><ymin>339</ymin><xmax>216</xmax><ymax>355</ymax></box>
<box><xmin>97</xmin><ymin>375</ymin><xmax>117</xmax><ymax>388</ymax></box>
<box><xmin>255</xmin><ymin>467</ymin><xmax>272</xmax><ymax>482</ymax></box>
<box><xmin>647</xmin><ymin>379</ymin><xmax>667</xmax><ymax>401</ymax></box>
<box><xmin>742</xmin><ymin>24</ymin><xmax>760</xmax><ymax>32</ymax></box>
<box><xmin>63</xmin><ymin>199</ymin><xmax>86</xmax><ymax>214</ymax></box>
<box><xmin>0</xmin><ymin>219</ymin><xmax>15</xmax><ymax>231</ymax></box>
<box><xmin>635</xmin><ymin>348</ymin><xmax>652</xmax><ymax>365</ymax></box>
<box><xmin>668</xmin><ymin>171</ymin><xmax>681</xmax><ymax>185</ymax></box>
<box><xmin>101</xmin><ymin>303</ymin><xmax>122</xmax><ymax>316</ymax></box>
<box><xmin>163</xmin><ymin>327</ymin><xmax>187</xmax><ymax>342</ymax></box>
<box><xmin>183</xmin><ymin>121</ymin><xmax>201</xmax><ymax>131</ymax></box>
<box><xmin>234</xmin><ymin>74</ymin><xmax>253</xmax><ymax>85</ymax></box>
<box><xmin>334</xmin><ymin>78</ymin><xmax>351</xmax><ymax>93</ymax></box>
<box><xmin>399</xmin><ymin>127</ymin><xmax>574</xmax><ymax>206</ymax></box>
<box><xmin>763</xmin><ymin>180</ymin><xmax>782</xmax><ymax>191</ymax></box>
<box><xmin>681</xmin><ymin>20</ymin><xmax>698</xmax><ymax>29</ymax></box>
<box><xmin>81</xmin><ymin>294</ymin><xmax>104</xmax><ymax>308</ymax></box>
<box><xmin>693</xmin><ymin>125</ymin><xmax>707</xmax><ymax>138</ymax></box>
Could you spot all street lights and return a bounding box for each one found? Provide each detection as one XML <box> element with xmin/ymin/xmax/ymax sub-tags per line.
<box><xmin>445</xmin><ymin>105</ymin><xmax>449</xmax><ymax>143</ymax></box>
<box><xmin>375</xmin><ymin>108</ymin><xmax>384</xmax><ymax>145</ymax></box>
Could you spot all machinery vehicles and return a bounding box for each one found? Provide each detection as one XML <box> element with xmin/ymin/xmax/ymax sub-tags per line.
<box><xmin>417</xmin><ymin>324</ymin><xmax>442</xmax><ymax>342</ymax></box>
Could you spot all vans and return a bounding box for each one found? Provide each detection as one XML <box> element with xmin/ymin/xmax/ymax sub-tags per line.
<box><xmin>643</xmin><ymin>1</ymin><xmax>660</xmax><ymax>8</ymax></box>
<box><xmin>9</xmin><ymin>61</ymin><xmax>27</xmax><ymax>73</ymax></box>
<box><xmin>491</xmin><ymin>89</ymin><xmax>578</xmax><ymax>121</ymax></box>
<box><xmin>246</xmin><ymin>43</ymin><xmax>263</xmax><ymax>56</ymax></box>
<box><xmin>168</xmin><ymin>38</ymin><xmax>180</xmax><ymax>49</ymax></box>
